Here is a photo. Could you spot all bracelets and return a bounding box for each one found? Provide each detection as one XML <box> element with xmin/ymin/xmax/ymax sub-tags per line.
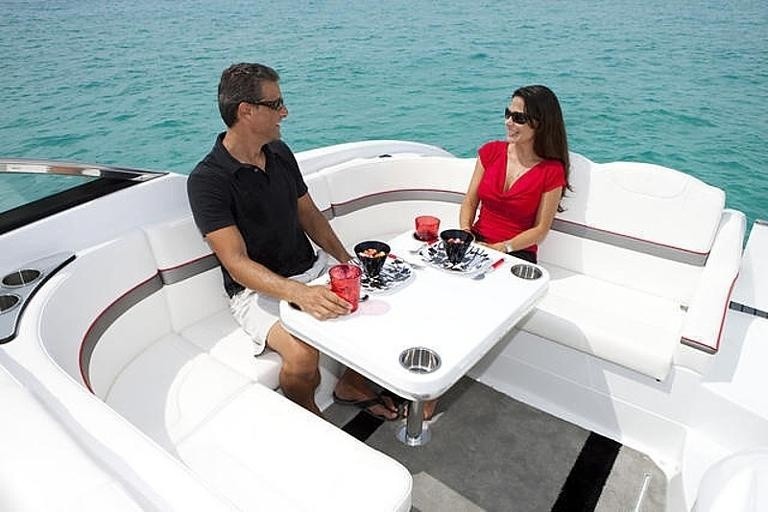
<box><xmin>505</xmin><ymin>239</ymin><xmax>513</xmax><ymax>253</ymax></box>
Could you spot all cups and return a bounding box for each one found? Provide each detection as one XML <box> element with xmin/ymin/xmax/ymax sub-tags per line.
<box><xmin>440</xmin><ymin>228</ymin><xmax>474</xmax><ymax>265</ymax></box>
<box><xmin>415</xmin><ymin>215</ymin><xmax>441</xmax><ymax>242</ymax></box>
<box><xmin>329</xmin><ymin>263</ymin><xmax>362</xmax><ymax>315</ymax></box>
<box><xmin>353</xmin><ymin>240</ymin><xmax>391</xmax><ymax>279</ymax></box>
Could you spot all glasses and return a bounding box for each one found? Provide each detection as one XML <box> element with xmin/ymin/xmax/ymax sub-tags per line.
<box><xmin>239</xmin><ymin>98</ymin><xmax>283</xmax><ymax>112</ymax></box>
<box><xmin>505</xmin><ymin>107</ymin><xmax>527</xmax><ymax>124</ymax></box>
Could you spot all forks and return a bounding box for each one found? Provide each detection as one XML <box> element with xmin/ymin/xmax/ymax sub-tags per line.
<box><xmin>471</xmin><ymin>257</ymin><xmax>505</xmax><ymax>280</ymax></box>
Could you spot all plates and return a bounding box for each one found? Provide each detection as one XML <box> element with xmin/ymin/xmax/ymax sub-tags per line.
<box><xmin>418</xmin><ymin>240</ymin><xmax>491</xmax><ymax>275</ymax></box>
<box><xmin>345</xmin><ymin>254</ymin><xmax>415</xmax><ymax>294</ymax></box>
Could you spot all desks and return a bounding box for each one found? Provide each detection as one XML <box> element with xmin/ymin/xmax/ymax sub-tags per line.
<box><xmin>279</xmin><ymin>230</ymin><xmax>550</xmax><ymax>446</ymax></box>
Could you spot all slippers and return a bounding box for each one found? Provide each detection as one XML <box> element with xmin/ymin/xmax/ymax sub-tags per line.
<box><xmin>333</xmin><ymin>385</ymin><xmax>399</xmax><ymax>421</ymax></box>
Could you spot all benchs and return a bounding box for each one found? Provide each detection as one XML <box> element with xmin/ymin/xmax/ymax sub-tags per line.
<box><xmin>328</xmin><ymin>160</ymin><xmax>728</xmax><ymax>382</ymax></box>
<box><xmin>35</xmin><ymin>172</ymin><xmax>415</xmax><ymax>512</ymax></box>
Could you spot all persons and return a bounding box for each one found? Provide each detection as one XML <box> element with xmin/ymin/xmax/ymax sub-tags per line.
<box><xmin>183</xmin><ymin>62</ymin><xmax>399</xmax><ymax>422</ymax></box>
<box><xmin>397</xmin><ymin>84</ymin><xmax>574</xmax><ymax>420</ymax></box>
